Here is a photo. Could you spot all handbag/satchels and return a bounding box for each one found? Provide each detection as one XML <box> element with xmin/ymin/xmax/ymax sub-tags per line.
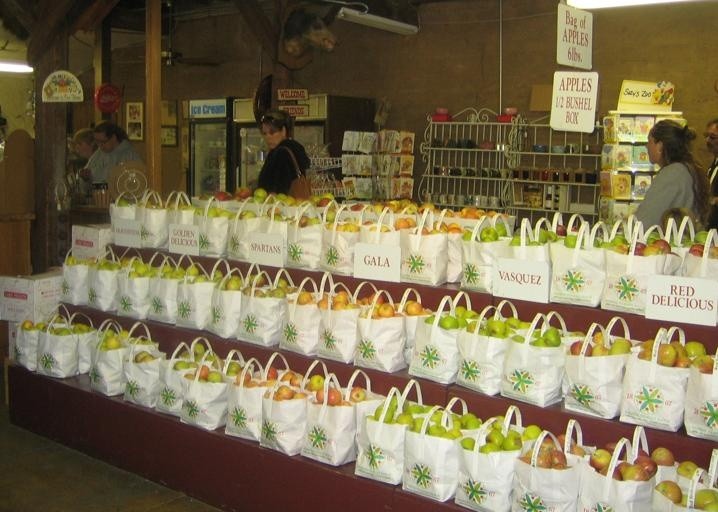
<box><xmin>457</xmin><ymin>210</ymin><xmax>718</xmax><ymax>512</ymax></box>
<box><xmin>18</xmin><ymin>192</ymin><xmax>230</xmax><ymax>432</ymax></box>
<box><xmin>225</xmin><ymin>194</ymin><xmax>462</xmax><ymax>503</ymax></box>
<box><xmin>288</xmin><ymin>178</ymin><xmax>311</xmax><ymax>200</ymax></box>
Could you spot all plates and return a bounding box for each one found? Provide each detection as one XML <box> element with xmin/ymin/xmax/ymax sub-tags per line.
<box><xmin>108</xmin><ymin>158</ymin><xmax>149</xmax><ymax>206</ymax></box>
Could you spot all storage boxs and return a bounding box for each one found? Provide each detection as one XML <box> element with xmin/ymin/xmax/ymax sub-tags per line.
<box><xmin>9</xmin><ymin>323</ymin><xmax>20</xmax><ymax>362</ymax></box>
<box><xmin>72</xmin><ymin>223</ymin><xmax>113</xmax><ymax>262</ymax></box>
<box><xmin>1</xmin><ymin>274</ymin><xmax>64</xmax><ymax>323</ymax></box>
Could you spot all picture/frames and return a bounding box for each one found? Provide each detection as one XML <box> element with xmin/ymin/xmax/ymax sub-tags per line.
<box><xmin>161</xmin><ymin>127</ymin><xmax>180</xmax><ymax>148</ymax></box>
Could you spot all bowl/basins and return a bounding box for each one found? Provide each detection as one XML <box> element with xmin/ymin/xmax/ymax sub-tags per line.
<box><xmin>534</xmin><ymin>142</ymin><xmax>574</xmax><ymax>154</ymax></box>
<box><xmin>432</xmin><ymin>107</ymin><xmax>450</xmax><ymax>122</ymax></box>
<box><xmin>496</xmin><ymin>107</ymin><xmax>519</xmax><ymax>123</ymax></box>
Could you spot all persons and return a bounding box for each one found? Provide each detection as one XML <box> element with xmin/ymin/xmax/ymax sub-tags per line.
<box><xmin>1</xmin><ymin>118</ymin><xmax>7</xmax><ymax>133</ymax></box>
<box><xmin>256</xmin><ymin>109</ymin><xmax>310</xmax><ymax>196</ymax></box>
<box><xmin>630</xmin><ymin>119</ymin><xmax>708</xmax><ymax>237</ymax></box>
<box><xmin>74</xmin><ymin>128</ymin><xmax>108</xmax><ymax>191</ymax></box>
<box><xmin>79</xmin><ymin>121</ymin><xmax>145</xmax><ymax>196</ymax></box>
<box><xmin>0</xmin><ymin>129</ymin><xmax>7</xmax><ymax>162</ymax></box>
<box><xmin>704</xmin><ymin>120</ymin><xmax>718</xmax><ymax>233</ymax></box>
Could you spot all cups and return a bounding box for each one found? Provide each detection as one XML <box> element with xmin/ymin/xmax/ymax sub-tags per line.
<box><xmin>435</xmin><ymin>164</ymin><xmax>597</xmax><ymax>183</ymax></box>
<box><xmin>245</xmin><ymin>151</ymin><xmax>267</xmax><ymax>161</ymax></box>
<box><xmin>425</xmin><ymin>192</ymin><xmax>499</xmax><ymax>207</ymax></box>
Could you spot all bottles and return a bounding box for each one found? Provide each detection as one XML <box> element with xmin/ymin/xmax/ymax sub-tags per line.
<box><xmin>547</xmin><ymin>185</ymin><xmax>559</xmax><ymax>210</ymax></box>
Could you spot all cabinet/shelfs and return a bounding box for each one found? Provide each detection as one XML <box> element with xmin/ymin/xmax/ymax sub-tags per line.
<box><xmin>415</xmin><ymin>106</ymin><xmax>603</xmax><ymax>229</ymax></box>
<box><xmin>592</xmin><ymin>110</ymin><xmax>689</xmax><ymax>232</ymax></box>
<box><xmin>305</xmin><ymin>155</ymin><xmax>349</xmax><ymax>200</ymax></box>
<box><xmin>8</xmin><ymin>242</ymin><xmax>718</xmax><ymax>511</ymax></box>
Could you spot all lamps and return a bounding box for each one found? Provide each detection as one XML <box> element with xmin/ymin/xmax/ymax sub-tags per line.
<box><xmin>322</xmin><ymin>1</ymin><xmax>421</xmax><ymax>37</ymax></box>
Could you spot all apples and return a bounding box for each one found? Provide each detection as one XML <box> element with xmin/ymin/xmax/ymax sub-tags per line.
<box><xmin>22</xmin><ymin>186</ymin><xmax>718</xmax><ymax>512</ymax></box>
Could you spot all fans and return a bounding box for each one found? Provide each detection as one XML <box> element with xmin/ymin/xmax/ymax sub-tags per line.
<box><xmin>138</xmin><ymin>0</ymin><xmax>223</xmax><ymax>70</ymax></box>
